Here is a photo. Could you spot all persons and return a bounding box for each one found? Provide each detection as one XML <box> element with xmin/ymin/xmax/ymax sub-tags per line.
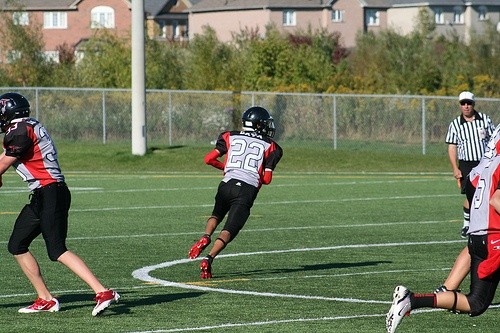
<box><xmin>445</xmin><ymin>91</ymin><xmax>495</xmax><ymax>240</ymax></box>
<box><xmin>189</xmin><ymin>106</ymin><xmax>283</xmax><ymax>279</ymax></box>
<box><xmin>0</xmin><ymin>93</ymin><xmax>121</xmax><ymax>316</ymax></box>
<box><xmin>385</xmin><ymin>124</ymin><xmax>500</xmax><ymax>333</ymax></box>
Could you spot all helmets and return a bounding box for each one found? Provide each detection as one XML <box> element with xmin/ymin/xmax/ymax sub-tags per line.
<box><xmin>242</xmin><ymin>106</ymin><xmax>277</xmax><ymax>139</ymax></box>
<box><xmin>0</xmin><ymin>93</ymin><xmax>31</xmax><ymax>131</ymax></box>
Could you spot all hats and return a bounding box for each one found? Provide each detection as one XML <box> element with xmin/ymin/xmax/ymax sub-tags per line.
<box><xmin>459</xmin><ymin>91</ymin><xmax>475</xmax><ymax>102</ymax></box>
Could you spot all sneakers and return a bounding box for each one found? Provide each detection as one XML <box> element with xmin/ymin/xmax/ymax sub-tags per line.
<box><xmin>199</xmin><ymin>258</ymin><xmax>212</xmax><ymax>279</ymax></box>
<box><xmin>461</xmin><ymin>226</ymin><xmax>469</xmax><ymax>238</ymax></box>
<box><xmin>18</xmin><ymin>295</ymin><xmax>60</xmax><ymax>313</ymax></box>
<box><xmin>433</xmin><ymin>286</ymin><xmax>448</xmax><ymax>294</ymax></box>
<box><xmin>385</xmin><ymin>285</ymin><xmax>412</xmax><ymax>333</ymax></box>
<box><xmin>188</xmin><ymin>235</ymin><xmax>211</xmax><ymax>259</ymax></box>
<box><xmin>92</xmin><ymin>289</ymin><xmax>121</xmax><ymax>316</ymax></box>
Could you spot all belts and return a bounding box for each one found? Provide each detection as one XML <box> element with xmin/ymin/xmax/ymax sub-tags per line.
<box><xmin>236</xmin><ymin>182</ymin><xmax>242</xmax><ymax>186</ymax></box>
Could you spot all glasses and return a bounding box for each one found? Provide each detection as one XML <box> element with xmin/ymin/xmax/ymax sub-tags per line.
<box><xmin>460</xmin><ymin>100</ymin><xmax>473</xmax><ymax>106</ymax></box>
